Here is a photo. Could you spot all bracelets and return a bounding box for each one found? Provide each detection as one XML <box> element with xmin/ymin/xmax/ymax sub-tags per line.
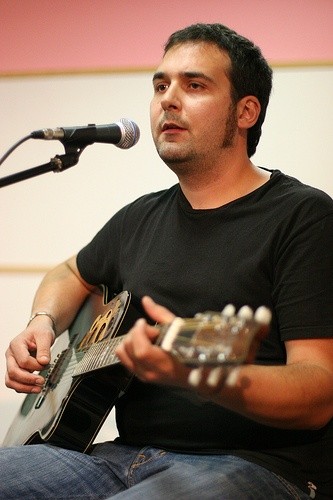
<box><xmin>27</xmin><ymin>312</ymin><xmax>57</xmax><ymax>331</ymax></box>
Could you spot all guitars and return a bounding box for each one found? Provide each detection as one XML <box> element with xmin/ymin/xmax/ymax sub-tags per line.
<box><xmin>2</xmin><ymin>283</ymin><xmax>287</xmax><ymax>455</ymax></box>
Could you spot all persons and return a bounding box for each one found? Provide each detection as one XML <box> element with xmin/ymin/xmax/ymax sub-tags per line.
<box><xmin>0</xmin><ymin>24</ymin><xmax>333</xmax><ymax>500</ymax></box>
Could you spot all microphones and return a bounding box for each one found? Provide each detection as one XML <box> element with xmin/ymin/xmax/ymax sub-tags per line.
<box><xmin>32</xmin><ymin>118</ymin><xmax>140</xmax><ymax>149</ymax></box>
